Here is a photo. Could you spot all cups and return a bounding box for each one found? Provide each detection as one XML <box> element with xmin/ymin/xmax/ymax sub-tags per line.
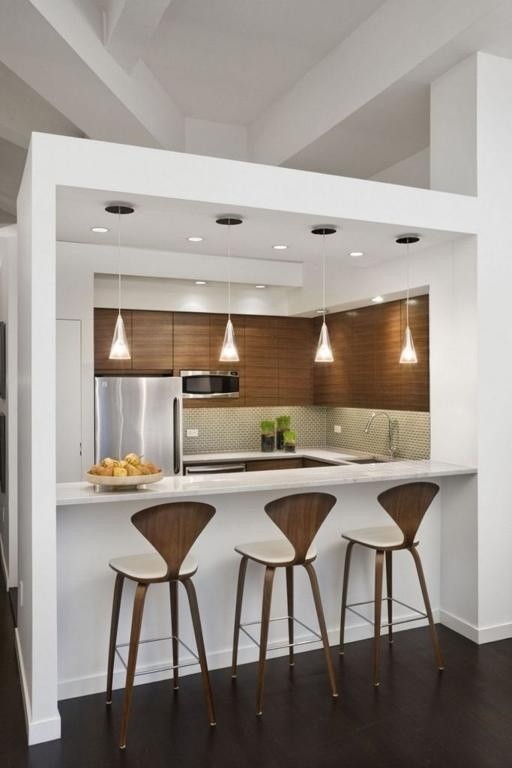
<box><xmin>278</xmin><ymin>431</ymin><xmax>284</xmax><ymax>450</ymax></box>
<box><xmin>261</xmin><ymin>434</ymin><xmax>275</xmax><ymax>452</ymax></box>
<box><xmin>284</xmin><ymin>442</ymin><xmax>295</xmax><ymax>451</ymax></box>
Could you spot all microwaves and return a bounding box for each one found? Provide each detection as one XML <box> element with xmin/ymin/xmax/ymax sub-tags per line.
<box><xmin>179</xmin><ymin>370</ymin><xmax>240</xmax><ymax>399</ymax></box>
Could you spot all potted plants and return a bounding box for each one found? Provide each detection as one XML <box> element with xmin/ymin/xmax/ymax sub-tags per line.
<box><xmin>259</xmin><ymin>420</ymin><xmax>275</xmax><ymax>452</ymax></box>
<box><xmin>281</xmin><ymin>431</ymin><xmax>296</xmax><ymax>452</ymax></box>
<box><xmin>276</xmin><ymin>415</ymin><xmax>290</xmax><ymax>449</ymax></box>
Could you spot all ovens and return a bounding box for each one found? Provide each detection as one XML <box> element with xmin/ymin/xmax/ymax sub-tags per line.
<box><xmin>185</xmin><ymin>464</ymin><xmax>247</xmax><ymax>476</ymax></box>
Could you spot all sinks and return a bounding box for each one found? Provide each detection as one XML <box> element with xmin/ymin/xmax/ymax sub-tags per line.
<box><xmin>337</xmin><ymin>454</ymin><xmax>391</xmax><ymax>464</ymax></box>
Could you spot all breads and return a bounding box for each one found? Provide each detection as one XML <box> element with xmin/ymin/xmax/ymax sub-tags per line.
<box><xmin>90</xmin><ymin>453</ymin><xmax>157</xmax><ymax>476</ymax></box>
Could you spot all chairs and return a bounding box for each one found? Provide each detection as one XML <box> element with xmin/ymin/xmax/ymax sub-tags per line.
<box><xmin>105</xmin><ymin>499</ymin><xmax>216</xmax><ymax>751</ymax></box>
<box><xmin>224</xmin><ymin>491</ymin><xmax>339</xmax><ymax>718</ymax></box>
<box><xmin>341</xmin><ymin>479</ymin><xmax>446</xmax><ymax>687</ymax></box>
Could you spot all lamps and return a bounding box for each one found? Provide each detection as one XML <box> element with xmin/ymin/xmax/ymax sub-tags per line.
<box><xmin>308</xmin><ymin>228</ymin><xmax>337</xmax><ymax>364</ymax></box>
<box><xmin>217</xmin><ymin>217</ymin><xmax>241</xmax><ymax>363</ymax></box>
<box><xmin>395</xmin><ymin>235</ymin><xmax>418</xmax><ymax>365</ymax></box>
<box><xmin>105</xmin><ymin>205</ymin><xmax>136</xmax><ymax>359</ymax></box>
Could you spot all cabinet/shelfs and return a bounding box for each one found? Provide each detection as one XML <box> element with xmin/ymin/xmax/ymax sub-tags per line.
<box><xmin>174</xmin><ymin>311</ymin><xmax>246</xmax><ymax>407</ymax></box>
<box><xmin>375</xmin><ymin>294</ymin><xmax>429</xmax><ymax>413</ymax></box>
<box><xmin>245</xmin><ymin>315</ymin><xmax>312</xmax><ymax>404</ymax></box>
<box><xmin>310</xmin><ymin>305</ymin><xmax>376</xmax><ymax>408</ymax></box>
<box><xmin>94</xmin><ymin>307</ymin><xmax>173</xmax><ymax>374</ymax></box>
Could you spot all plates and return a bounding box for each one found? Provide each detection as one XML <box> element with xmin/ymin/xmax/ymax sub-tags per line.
<box><xmin>86</xmin><ymin>469</ymin><xmax>162</xmax><ymax>486</ymax></box>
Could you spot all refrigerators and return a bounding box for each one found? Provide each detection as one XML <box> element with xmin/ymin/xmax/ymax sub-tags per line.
<box><xmin>93</xmin><ymin>376</ymin><xmax>183</xmax><ymax>480</ymax></box>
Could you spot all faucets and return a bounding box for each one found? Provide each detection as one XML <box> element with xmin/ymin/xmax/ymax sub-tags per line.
<box><xmin>365</xmin><ymin>412</ymin><xmax>391</xmax><ymax>447</ymax></box>
